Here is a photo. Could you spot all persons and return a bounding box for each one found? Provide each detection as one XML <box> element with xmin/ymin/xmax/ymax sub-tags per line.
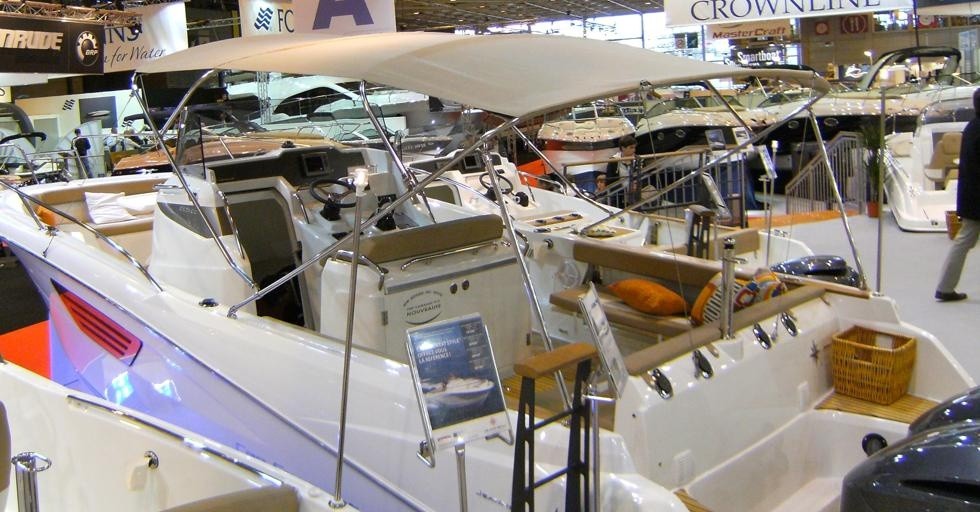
<box><xmin>111</xmin><ymin>127</ymin><xmax>118</xmax><ymax>135</ymax></box>
<box><xmin>932</xmin><ymin>86</ymin><xmax>979</xmax><ymax>301</ymax></box>
<box><xmin>604</xmin><ymin>135</ymin><xmax>642</xmax><ymax>207</ymax></box>
<box><xmin>128</xmin><ymin>129</ymin><xmax>143</xmax><ymax>150</ymax></box>
<box><xmin>71</xmin><ymin>129</ymin><xmax>93</xmax><ymax>178</ymax></box>
<box><xmin>122</xmin><ymin>130</ymin><xmax>139</xmax><ymax>151</ymax></box>
<box><xmin>538</xmin><ymin>174</ymin><xmax>555</xmax><ymax>190</ymax></box>
<box><xmin>594</xmin><ymin>173</ymin><xmax>605</xmax><ymax>203</ymax></box>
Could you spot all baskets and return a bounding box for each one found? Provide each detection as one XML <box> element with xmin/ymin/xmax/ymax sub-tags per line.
<box><xmin>830</xmin><ymin>324</ymin><xmax>917</xmax><ymax>405</ymax></box>
<box><xmin>946</xmin><ymin>210</ymin><xmax>962</xmax><ymax>240</ymax></box>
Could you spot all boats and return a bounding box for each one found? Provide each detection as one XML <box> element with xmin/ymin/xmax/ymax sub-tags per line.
<box><xmin>0</xmin><ymin>194</ymin><xmax>363</xmax><ymax>512</ymax></box>
<box><xmin>0</xmin><ymin>43</ymin><xmax>980</xmax><ymax>289</ymax></box>
<box><xmin>0</xmin><ymin>30</ymin><xmax>979</xmax><ymax>512</ymax></box>
<box><xmin>424</xmin><ymin>377</ymin><xmax>495</xmax><ymax>410</ymax></box>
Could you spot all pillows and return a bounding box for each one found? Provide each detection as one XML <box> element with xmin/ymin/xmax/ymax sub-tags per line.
<box><xmin>606</xmin><ymin>270</ymin><xmax>787</xmax><ymax>326</ymax></box>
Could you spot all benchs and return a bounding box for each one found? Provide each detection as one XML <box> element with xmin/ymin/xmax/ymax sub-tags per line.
<box><xmin>547</xmin><ymin>240</ymin><xmax>755</xmax><ymax>338</ymax></box>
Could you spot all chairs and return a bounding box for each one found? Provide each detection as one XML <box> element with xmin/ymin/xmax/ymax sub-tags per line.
<box><xmin>921</xmin><ymin>132</ymin><xmax>963</xmax><ymax>182</ymax></box>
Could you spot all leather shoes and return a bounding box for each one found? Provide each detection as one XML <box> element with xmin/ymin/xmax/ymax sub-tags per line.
<box><xmin>936</xmin><ymin>290</ymin><xmax>966</xmax><ymax>301</ymax></box>
